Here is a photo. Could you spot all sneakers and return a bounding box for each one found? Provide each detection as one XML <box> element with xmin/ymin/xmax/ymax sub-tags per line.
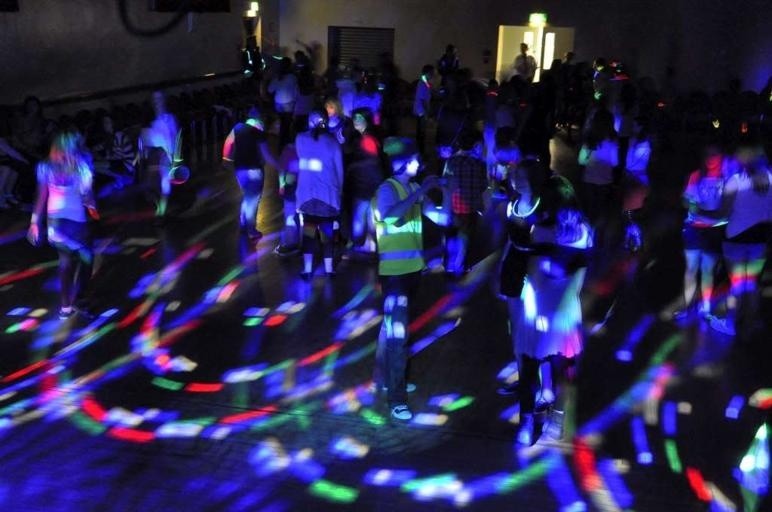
<box><xmin>392</xmin><ymin>404</ymin><xmax>412</xmax><ymax>421</ymax></box>
<box><xmin>369</xmin><ymin>381</ymin><xmax>416</xmax><ymax>392</ymax></box>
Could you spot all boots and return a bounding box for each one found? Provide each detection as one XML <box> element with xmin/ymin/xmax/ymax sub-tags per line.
<box><xmin>516</xmin><ymin>412</ymin><xmax>534</xmax><ymax>446</ymax></box>
<box><xmin>536</xmin><ymin>404</ymin><xmax>565</xmax><ymax>439</ymax></box>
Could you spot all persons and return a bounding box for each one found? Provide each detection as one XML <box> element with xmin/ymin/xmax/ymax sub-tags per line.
<box><xmin>0</xmin><ymin>42</ymin><xmax>772</xmax><ymax>447</ymax></box>
